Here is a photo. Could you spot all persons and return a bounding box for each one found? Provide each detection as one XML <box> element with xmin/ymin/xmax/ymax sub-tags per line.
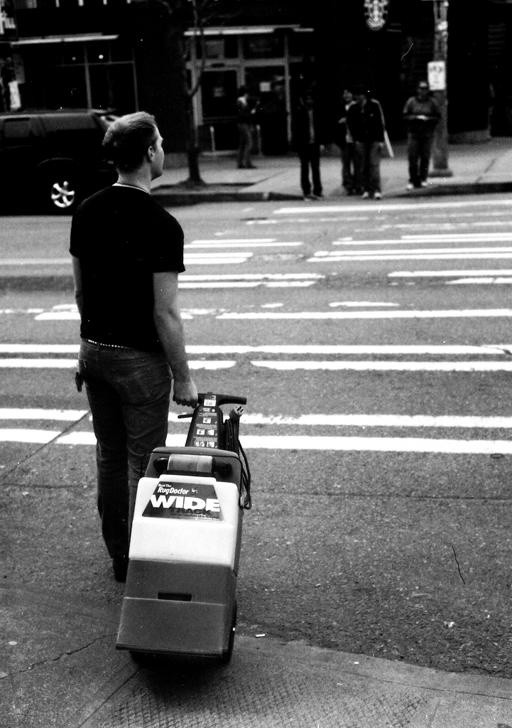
<box><xmin>235</xmin><ymin>80</ymin><xmax>442</xmax><ymax>202</ymax></box>
<box><xmin>67</xmin><ymin>109</ymin><xmax>200</xmax><ymax>584</ymax></box>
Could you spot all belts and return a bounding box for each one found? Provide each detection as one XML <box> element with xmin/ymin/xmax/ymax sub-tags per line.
<box><xmin>80</xmin><ymin>339</ymin><xmax>151</xmax><ymax>360</ymax></box>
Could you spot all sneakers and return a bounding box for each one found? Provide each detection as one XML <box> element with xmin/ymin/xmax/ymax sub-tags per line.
<box><xmin>238</xmin><ymin>163</ymin><xmax>257</xmax><ymax>169</ymax></box>
<box><xmin>404</xmin><ymin>179</ymin><xmax>433</xmax><ymax>190</ymax></box>
<box><xmin>304</xmin><ymin>188</ymin><xmax>324</xmax><ymax>199</ymax></box>
<box><xmin>346</xmin><ymin>188</ymin><xmax>384</xmax><ymax>200</ymax></box>
<box><xmin>111</xmin><ymin>552</ymin><xmax>129</xmax><ymax>582</ymax></box>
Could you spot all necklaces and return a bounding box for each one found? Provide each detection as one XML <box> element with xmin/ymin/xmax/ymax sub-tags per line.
<box><xmin>114</xmin><ymin>179</ymin><xmax>150</xmax><ymax>193</ymax></box>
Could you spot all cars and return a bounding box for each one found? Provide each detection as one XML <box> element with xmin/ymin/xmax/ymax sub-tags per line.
<box><xmin>0</xmin><ymin>107</ymin><xmax>119</xmax><ymax>213</ymax></box>
